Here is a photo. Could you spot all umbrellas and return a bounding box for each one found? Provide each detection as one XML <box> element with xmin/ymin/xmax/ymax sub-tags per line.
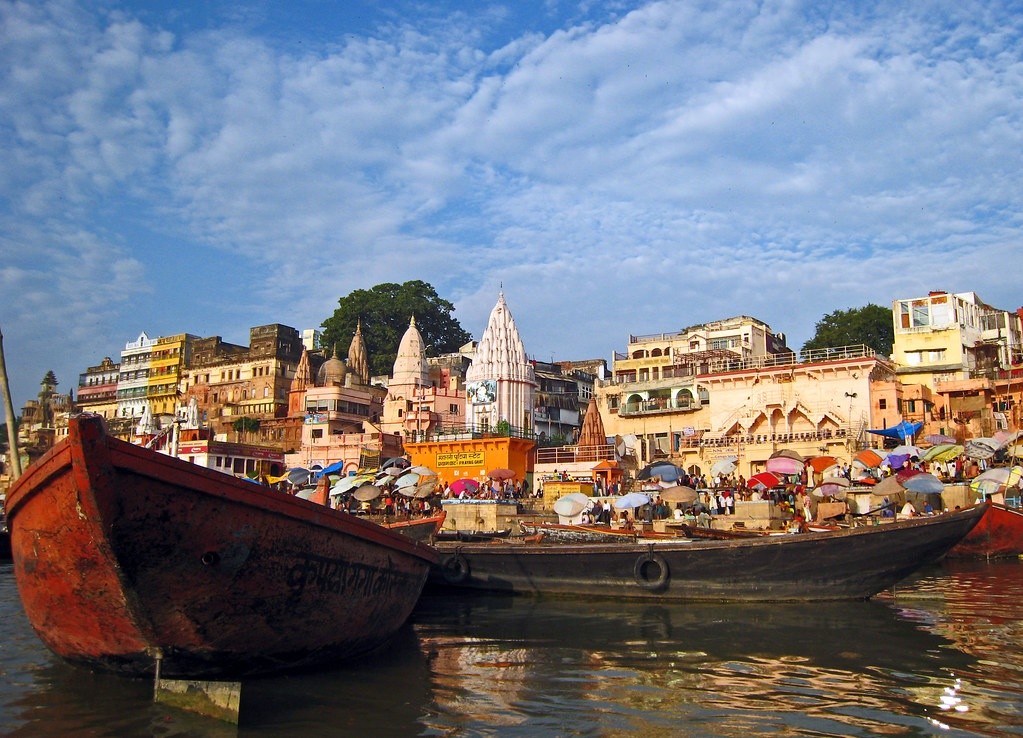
<box><xmin>554</xmin><ymin>492</ymin><xmax>589</xmax><ymax>517</ymax></box>
<box><xmin>615</xmin><ymin>492</ymin><xmax>650</xmax><ymax>509</ymax></box>
<box><xmin>636</xmin><ymin>461</ymin><xmax>686</xmax><ymax>482</ymax></box>
<box><xmin>710</xmin><ymin>456</ymin><xmax>738</xmax><ymax>479</ymax></box>
<box><xmin>747</xmin><ymin>429</ymin><xmax>1023</xmax><ymax>497</ymax></box>
<box><xmin>488</xmin><ymin>468</ymin><xmax>515</xmax><ymax>480</ymax></box>
<box><xmin>938</xmin><ymin>500</ymin><xmax>1023</xmax><ymax>559</ymax></box>
<box><xmin>240</xmin><ymin>458</ymin><xmax>438</xmax><ymax>500</ymax></box>
<box><xmin>659</xmin><ymin>486</ymin><xmax>698</xmax><ymax>503</ymax></box>
<box><xmin>450</xmin><ymin>479</ymin><xmax>479</xmax><ymax>497</ymax></box>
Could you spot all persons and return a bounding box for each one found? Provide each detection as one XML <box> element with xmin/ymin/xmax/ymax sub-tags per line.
<box><xmin>439</xmin><ymin>481</ymin><xmax>449</xmax><ymax>498</ymax></box>
<box><xmin>756</xmin><ymin>455</ymin><xmax>1023</xmax><ymax>533</ymax></box>
<box><xmin>582</xmin><ymin>478</ymin><xmax>666</xmax><ymax>531</ymax></box>
<box><xmin>673</xmin><ymin>472</ymin><xmax>745</xmax><ymax>527</ymax></box>
<box><xmin>450</xmin><ymin>477</ymin><xmax>543</xmax><ymax>499</ymax></box>
<box><xmin>553</xmin><ymin>469</ymin><xmax>574</xmax><ymax>482</ymax></box>
<box><xmin>329</xmin><ymin>485</ymin><xmax>435</xmax><ymax>519</ymax></box>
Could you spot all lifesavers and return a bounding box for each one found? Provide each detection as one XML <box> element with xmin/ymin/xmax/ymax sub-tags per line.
<box><xmin>631</xmin><ymin>555</ymin><xmax>670</xmax><ymax>592</ymax></box>
<box><xmin>438</xmin><ymin>554</ymin><xmax>471</xmax><ymax>585</ymax></box>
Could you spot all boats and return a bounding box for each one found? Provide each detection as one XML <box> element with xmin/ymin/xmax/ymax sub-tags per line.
<box><xmin>377</xmin><ymin>508</ymin><xmax>448</xmax><ymax>545</ymax></box>
<box><xmin>432</xmin><ymin>527</ymin><xmax>515</xmax><ymax>542</ymax></box>
<box><xmin>431</xmin><ymin>496</ymin><xmax>998</xmax><ymax>604</ymax></box>
<box><xmin>938</xmin><ymin>499</ymin><xmax>1023</xmax><ymax>560</ymax></box>
<box><xmin>0</xmin><ymin>321</ymin><xmax>447</xmax><ymax>726</ymax></box>
